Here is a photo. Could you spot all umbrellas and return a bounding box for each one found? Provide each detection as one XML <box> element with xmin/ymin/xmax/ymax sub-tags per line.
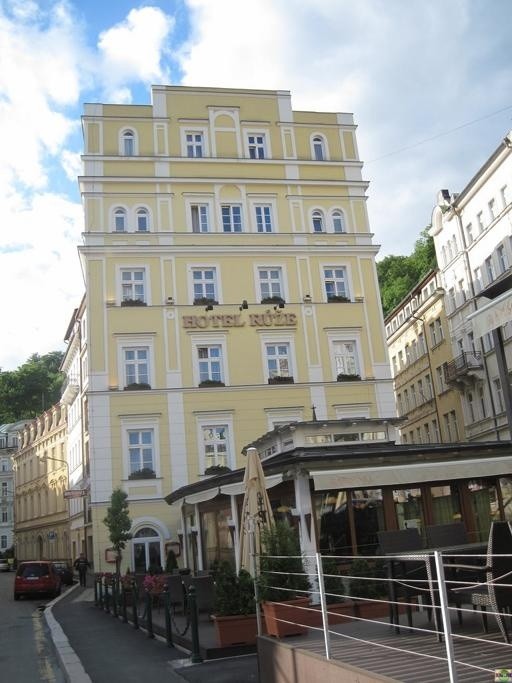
<box><xmin>235</xmin><ymin>443</ymin><xmax>284</xmax><ymax>637</ymax></box>
<box><xmin>164</xmin><ymin>549</ymin><xmax>179</xmax><ymax>574</ymax></box>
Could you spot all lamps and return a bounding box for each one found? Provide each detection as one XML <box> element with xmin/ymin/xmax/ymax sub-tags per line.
<box><xmin>200</xmin><ymin>300</ymin><xmax>286</xmax><ymax>312</ymax></box>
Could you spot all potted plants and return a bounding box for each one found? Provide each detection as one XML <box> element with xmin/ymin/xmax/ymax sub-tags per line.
<box><xmin>209</xmin><ymin>564</ymin><xmax>265</xmax><ymax>648</ymax></box>
<box><xmin>304</xmin><ymin>554</ymin><xmax>356</xmax><ymax>628</ymax></box>
<box><xmin>248</xmin><ymin>515</ymin><xmax>312</xmax><ymax>643</ymax></box>
<box><xmin>349</xmin><ymin>542</ymin><xmax>419</xmax><ymax>619</ymax></box>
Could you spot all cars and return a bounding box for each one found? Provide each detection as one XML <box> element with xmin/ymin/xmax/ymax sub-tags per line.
<box><xmin>14</xmin><ymin>559</ymin><xmax>62</xmax><ymax>601</ymax></box>
<box><xmin>52</xmin><ymin>561</ymin><xmax>74</xmax><ymax>585</ymax></box>
<box><xmin>0</xmin><ymin>558</ymin><xmax>10</xmax><ymax>570</ymax></box>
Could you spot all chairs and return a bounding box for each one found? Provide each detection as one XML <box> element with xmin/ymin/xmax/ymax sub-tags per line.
<box><xmin>374</xmin><ymin>526</ymin><xmax>432</xmax><ymax>634</ymax></box>
<box><xmin>467</xmin><ymin>519</ymin><xmax>512</xmax><ymax>646</ymax></box>
<box><xmin>126</xmin><ymin>560</ymin><xmax>229</xmax><ymax>619</ymax></box>
<box><xmin>424</xmin><ymin>521</ymin><xmax>479</xmax><ymax>625</ymax></box>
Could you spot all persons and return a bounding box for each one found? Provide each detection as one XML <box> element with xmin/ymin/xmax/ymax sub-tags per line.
<box><xmin>73</xmin><ymin>551</ymin><xmax>93</xmax><ymax>587</ymax></box>
